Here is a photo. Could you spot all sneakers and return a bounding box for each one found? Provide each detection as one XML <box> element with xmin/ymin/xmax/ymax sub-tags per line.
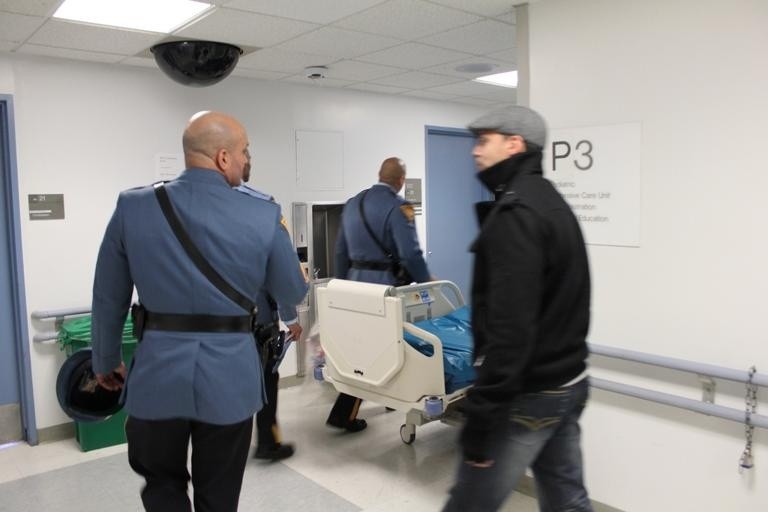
<box><xmin>325</xmin><ymin>416</ymin><xmax>368</xmax><ymax>433</ymax></box>
<box><xmin>253</xmin><ymin>442</ymin><xmax>296</xmax><ymax>460</ymax></box>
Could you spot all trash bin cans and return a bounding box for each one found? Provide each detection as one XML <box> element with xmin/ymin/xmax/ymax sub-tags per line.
<box><xmin>58</xmin><ymin>310</ymin><xmax>141</xmax><ymax>452</ymax></box>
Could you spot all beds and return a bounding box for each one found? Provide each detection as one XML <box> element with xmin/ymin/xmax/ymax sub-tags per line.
<box><xmin>315</xmin><ymin>279</ymin><xmax>478</xmax><ymax>444</ymax></box>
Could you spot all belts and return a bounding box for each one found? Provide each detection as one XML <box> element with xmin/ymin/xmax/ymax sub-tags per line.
<box><xmin>135</xmin><ymin>300</ymin><xmax>253</xmax><ymax>335</ymax></box>
<box><xmin>346</xmin><ymin>258</ymin><xmax>395</xmax><ymax>272</ymax></box>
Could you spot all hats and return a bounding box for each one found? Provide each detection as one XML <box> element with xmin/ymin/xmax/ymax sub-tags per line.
<box><xmin>466</xmin><ymin>105</ymin><xmax>547</xmax><ymax>149</ymax></box>
<box><xmin>57</xmin><ymin>348</ymin><xmax>127</xmax><ymax>424</ymax></box>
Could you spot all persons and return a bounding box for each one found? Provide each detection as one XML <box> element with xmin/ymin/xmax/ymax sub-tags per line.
<box><xmin>436</xmin><ymin>103</ymin><xmax>596</xmax><ymax>512</ymax></box>
<box><xmin>87</xmin><ymin>109</ymin><xmax>311</xmax><ymax>511</ymax></box>
<box><xmin>242</xmin><ymin>285</ymin><xmax>296</xmax><ymax>461</ymax></box>
<box><xmin>322</xmin><ymin>156</ymin><xmax>437</xmax><ymax>434</ymax></box>
